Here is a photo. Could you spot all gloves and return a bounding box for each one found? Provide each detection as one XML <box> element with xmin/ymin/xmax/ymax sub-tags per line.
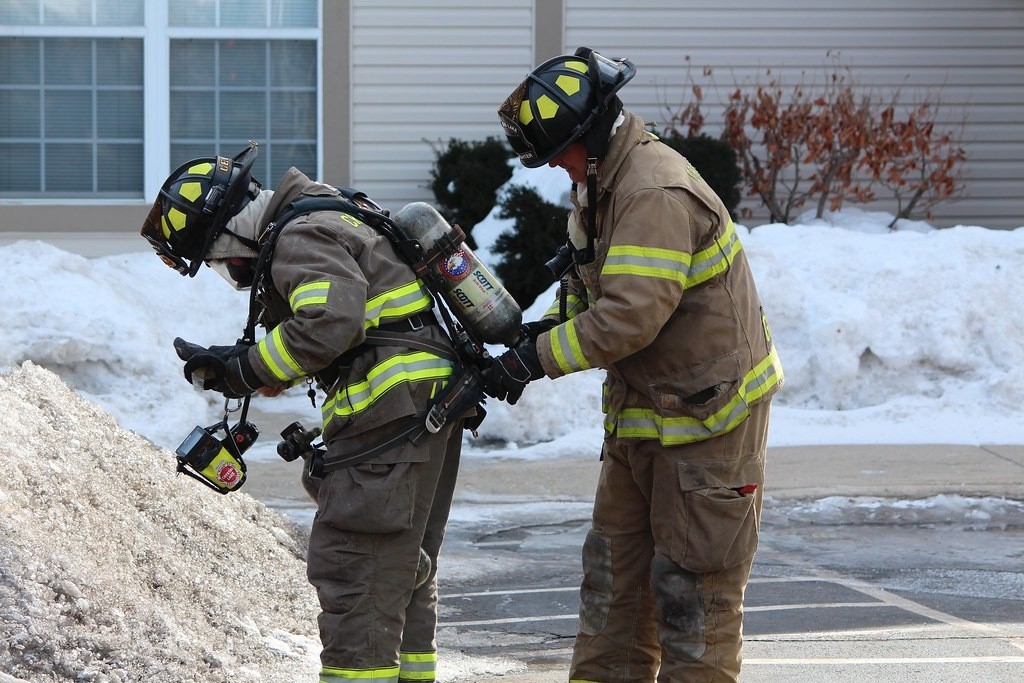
<box><xmin>486</xmin><ymin>343</ymin><xmax>544</xmax><ymax>405</ymax></box>
<box><xmin>513</xmin><ymin>319</ymin><xmax>559</xmax><ymax>348</ymax></box>
<box><xmin>174</xmin><ymin>336</ymin><xmax>264</xmax><ymax>399</ymax></box>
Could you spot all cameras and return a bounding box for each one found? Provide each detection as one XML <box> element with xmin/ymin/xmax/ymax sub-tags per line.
<box><xmin>175</xmin><ymin>420</ymin><xmax>260</xmax><ymax>495</ymax></box>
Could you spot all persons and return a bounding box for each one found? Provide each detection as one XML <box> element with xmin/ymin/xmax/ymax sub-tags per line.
<box><xmin>489</xmin><ymin>47</ymin><xmax>786</xmax><ymax>683</ymax></box>
<box><xmin>141</xmin><ymin>144</ymin><xmax>490</xmax><ymax>682</ymax></box>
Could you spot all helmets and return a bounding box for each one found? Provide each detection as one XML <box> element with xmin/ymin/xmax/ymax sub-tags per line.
<box><xmin>141</xmin><ymin>140</ymin><xmax>261</xmax><ymax>276</ymax></box>
<box><xmin>498</xmin><ymin>47</ymin><xmax>636</xmax><ymax>168</ymax></box>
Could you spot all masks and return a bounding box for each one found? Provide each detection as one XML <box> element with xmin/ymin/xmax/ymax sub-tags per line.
<box><xmin>227</xmin><ymin>262</ymin><xmax>253</xmax><ymax>282</ymax></box>
